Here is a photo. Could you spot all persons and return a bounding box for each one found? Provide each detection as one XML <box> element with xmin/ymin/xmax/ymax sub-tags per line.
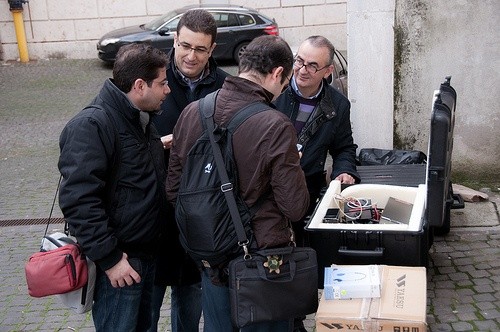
<box><xmin>166</xmin><ymin>35</ymin><xmax>310</xmax><ymax>332</ymax></box>
<box><xmin>57</xmin><ymin>40</ymin><xmax>171</xmax><ymax>332</ymax></box>
<box><xmin>273</xmin><ymin>36</ymin><xmax>361</xmax><ymax>287</ymax></box>
<box><xmin>137</xmin><ymin>9</ymin><xmax>231</xmax><ymax>332</ymax></box>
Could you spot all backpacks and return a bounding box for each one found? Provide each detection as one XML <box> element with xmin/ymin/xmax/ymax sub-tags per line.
<box><xmin>174</xmin><ymin>88</ymin><xmax>272</xmax><ymax>265</ymax></box>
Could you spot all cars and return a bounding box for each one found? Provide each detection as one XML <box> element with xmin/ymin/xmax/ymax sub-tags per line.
<box><xmin>97</xmin><ymin>4</ymin><xmax>280</xmax><ymax>66</ymax></box>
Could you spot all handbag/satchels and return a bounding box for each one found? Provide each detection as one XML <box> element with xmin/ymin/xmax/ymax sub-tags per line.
<box><xmin>39</xmin><ymin>231</ymin><xmax>96</xmax><ymax>313</ymax></box>
<box><xmin>25</xmin><ymin>243</ymin><xmax>89</xmax><ymax>297</ymax></box>
<box><xmin>229</xmin><ymin>246</ymin><xmax>318</xmax><ymax>329</ymax></box>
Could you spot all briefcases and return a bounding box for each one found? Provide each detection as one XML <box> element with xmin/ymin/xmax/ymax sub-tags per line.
<box><xmin>303</xmin><ymin>75</ymin><xmax>457</xmax><ymax>267</ymax></box>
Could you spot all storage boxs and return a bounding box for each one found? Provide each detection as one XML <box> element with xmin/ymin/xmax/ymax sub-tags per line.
<box><xmin>315</xmin><ymin>264</ymin><xmax>428</xmax><ymax>332</ymax></box>
<box><xmin>304</xmin><ymin>74</ymin><xmax>465</xmax><ymax>289</ymax></box>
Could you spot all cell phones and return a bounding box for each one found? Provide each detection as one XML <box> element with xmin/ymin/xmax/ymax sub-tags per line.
<box><xmin>323</xmin><ymin>208</ymin><xmax>340</xmax><ymax>220</ymax></box>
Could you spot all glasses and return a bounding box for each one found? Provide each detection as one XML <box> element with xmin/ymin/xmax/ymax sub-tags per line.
<box><xmin>176</xmin><ymin>38</ymin><xmax>209</xmax><ymax>53</ymax></box>
<box><xmin>282</xmin><ymin>73</ymin><xmax>291</xmax><ymax>88</ymax></box>
<box><xmin>134</xmin><ymin>80</ymin><xmax>168</xmax><ymax>88</ymax></box>
<box><xmin>293</xmin><ymin>58</ymin><xmax>329</xmax><ymax>73</ymax></box>
